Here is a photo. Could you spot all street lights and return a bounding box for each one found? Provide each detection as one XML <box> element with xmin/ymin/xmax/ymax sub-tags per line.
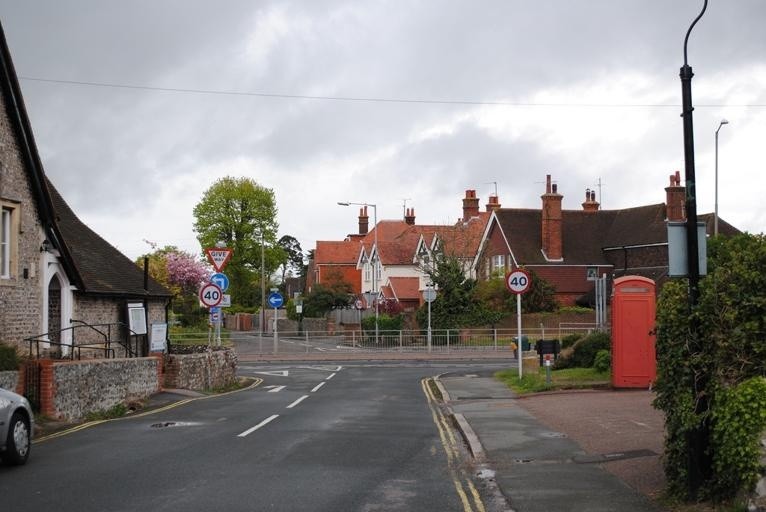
<box><xmin>336</xmin><ymin>200</ymin><xmax>381</xmax><ymax>348</ymax></box>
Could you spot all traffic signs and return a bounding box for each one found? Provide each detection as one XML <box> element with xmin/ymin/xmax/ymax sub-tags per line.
<box><xmin>200</xmin><ymin>282</ymin><xmax>224</xmax><ymax>308</ymax></box>
<box><xmin>713</xmin><ymin>117</ymin><xmax>730</xmax><ymax>240</ymax></box>
<box><xmin>504</xmin><ymin>268</ymin><xmax>534</xmax><ymax>297</ymax></box>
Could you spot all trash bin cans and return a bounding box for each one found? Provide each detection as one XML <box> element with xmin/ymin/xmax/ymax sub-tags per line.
<box><xmin>511</xmin><ymin>336</ymin><xmax>529</xmax><ymax>359</ymax></box>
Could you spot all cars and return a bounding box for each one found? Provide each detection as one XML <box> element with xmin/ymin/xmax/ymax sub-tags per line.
<box><xmin>0</xmin><ymin>386</ymin><xmax>36</xmax><ymax>469</ymax></box>
